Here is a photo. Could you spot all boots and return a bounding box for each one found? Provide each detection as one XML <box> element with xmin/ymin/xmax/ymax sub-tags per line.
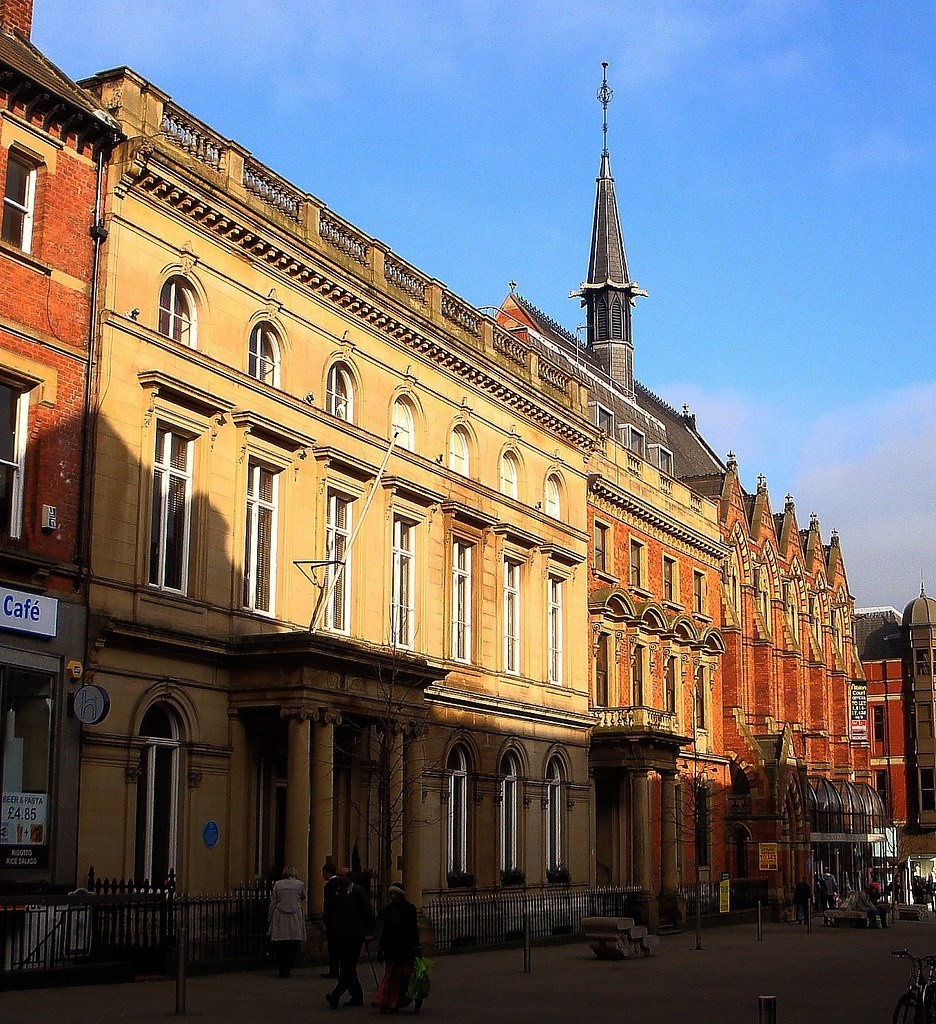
<box><xmin>344</xmin><ymin>977</ymin><xmax>364</xmax><ymax>1006</ymax></box>
<box><xmin>325</xmin><ymin>983</ymin><xmax>346</xmax><ymax>1010</ymax></box>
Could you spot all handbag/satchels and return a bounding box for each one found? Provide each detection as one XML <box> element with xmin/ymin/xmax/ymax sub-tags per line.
<box><xmin>406</xmin><ymin>956</ymin><xmax>437</xmax><ymax>1000</ymax></box>
<box><xmin>262</xmin><ymin>934</ymin><xmax>278</xmax><ymax>963</ymax></box>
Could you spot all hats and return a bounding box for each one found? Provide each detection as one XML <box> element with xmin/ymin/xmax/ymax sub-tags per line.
<box><xmin>389</xmin><ymin>882</ymin><xmax>407</xmax><ymax>896</ymax></box>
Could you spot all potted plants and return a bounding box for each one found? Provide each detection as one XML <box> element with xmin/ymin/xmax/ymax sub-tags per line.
<box><xmin>545</xmin><ymin>868</ymin><xmax>571</xmax><ymax>884</ymax></box>
<box><xmin>447</xmin><ymin>871</ymin><xmax>476</xmax><ymax>887</ymax></box>
<box><xmin>501</xmin><ymin>868</ymin><xmax>528</xmax><ymax>886</ymax></box>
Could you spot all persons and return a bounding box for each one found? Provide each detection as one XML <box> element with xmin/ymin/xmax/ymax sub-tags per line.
<box><xmin>321</xmin><ymin>863</ymin><xmax>354</xmax><ymax>979</ymax></box>
<box><xmin>377</xmin><ymin>882</ymin><xmax>423</xmax><ymax>1012</ymax></box>
<box><xmin>817</xmin><ymin>867</ymin><xmax>839</xmax><ymax>926</ymax></box>
<box><xmin>338</xmin><ymin>866</ymin><xmax>351</xmax><ymax>876</ymax></box>
<box><xmin>353</xmin><ymin>868</ymin><xmax>378</xmax><ymax>899</ymax></box>
<box><xmin>268</xmin><ymin>864</ymin><xmax>306</xmax><ymax>978</ymax></box>
<box><xmin>846</xmin><ymin>874</ymin><xmax>936</xmax><ymax>929</ymax></box>
<box><xmin>356</xmin><ymin>867</ymin><xmax>369</xmax><ymax>878</ymax></box>
<box><xmin>326</xmin><ymin>878</ymin><xmax>376</xmax><ymax>1009</ymax></box>
<box><xmin>793</xmin><ymin>876</ymin><xmax>813</xmax><ymax>925</ymax></box>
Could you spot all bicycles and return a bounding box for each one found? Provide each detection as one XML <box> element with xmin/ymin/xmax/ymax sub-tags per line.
<box><xmin>889</xmin><ymin>947</ymin><xmax>936</xmax><ymax>1024</ymax></box>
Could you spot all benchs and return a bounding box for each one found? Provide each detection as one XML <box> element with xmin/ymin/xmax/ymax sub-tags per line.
<box><xmin>823</xmin><ymin>908</ymin><xmax>870</xmax><ymax>928</ymax></box>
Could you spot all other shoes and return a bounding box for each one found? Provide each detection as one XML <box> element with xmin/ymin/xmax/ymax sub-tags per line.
<box><xmin>277</xmin><ymin>968</ymin><xmax>292</xmax><ymax>979</ymax></box>
<box><xmin>321</xmin><ymin>972</ymin><xmax>339</xmax><ymax>978</ymax></box>
<box><xmin>413</xmin><ymin>999</ymin><xmax>425</xmax><ymax>1011</ymax></box>
